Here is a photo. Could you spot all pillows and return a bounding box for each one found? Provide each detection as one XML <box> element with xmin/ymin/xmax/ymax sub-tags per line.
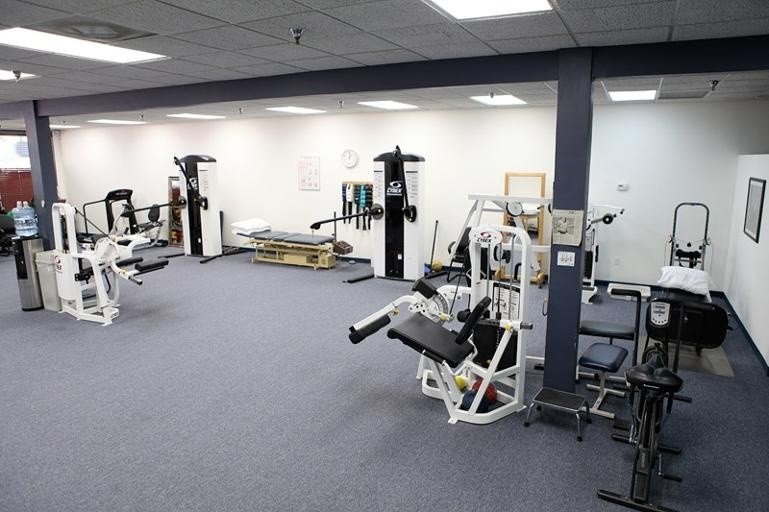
<box><xmin>657</xmin><ymin>266</ymin><xmax>711</xmax><ymax>293</ymax></box>
<box><xmin>231</xmin><ymin>219</ymin><xmax>271</xmax><ymax>236</ymax></box>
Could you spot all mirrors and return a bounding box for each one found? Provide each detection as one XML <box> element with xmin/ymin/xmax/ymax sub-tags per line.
<box><xmin>669</xmin><ymin>202</ymin><xmax>711</xmax><ymax>271</ymax></box>
<box><xmin>501</xmin><ymin>171</ymin><xmax>546</xmax><ymax>261</ymax></box>
<box><xmin>169</xmin><ymin>176</ymin><xmax>180</xmax><ymax>245</ymax></box>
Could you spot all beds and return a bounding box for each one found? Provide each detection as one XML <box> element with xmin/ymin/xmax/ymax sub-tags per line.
<box><xmin>230</xmin><ymin>219</ymin><xmax>338</xmax><ymax>271</ymax></box>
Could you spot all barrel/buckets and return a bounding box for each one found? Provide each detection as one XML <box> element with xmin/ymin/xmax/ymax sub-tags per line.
<box><xmin>11</xmin><ymin>207</ymin><xmax>39</xmax><ymax>238</ymax></box>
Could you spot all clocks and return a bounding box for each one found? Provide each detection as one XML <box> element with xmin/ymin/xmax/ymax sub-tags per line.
<box><xmin>340</xmin><ymin>149</ymin><xmax>359</xmax><ymax>167</ymax></box>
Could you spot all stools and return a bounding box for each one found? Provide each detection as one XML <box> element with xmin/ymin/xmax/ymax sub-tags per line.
<box><xmin>525</xmin><ymin>387</ymin><xmax>592</xmax><ymax>440</ymax></box>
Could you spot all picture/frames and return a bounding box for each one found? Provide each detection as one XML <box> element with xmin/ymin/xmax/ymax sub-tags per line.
<box><xmin>743</xmin><ymin>177</ymin><xmax>765</xmax><ymax>242</ymax></box>
